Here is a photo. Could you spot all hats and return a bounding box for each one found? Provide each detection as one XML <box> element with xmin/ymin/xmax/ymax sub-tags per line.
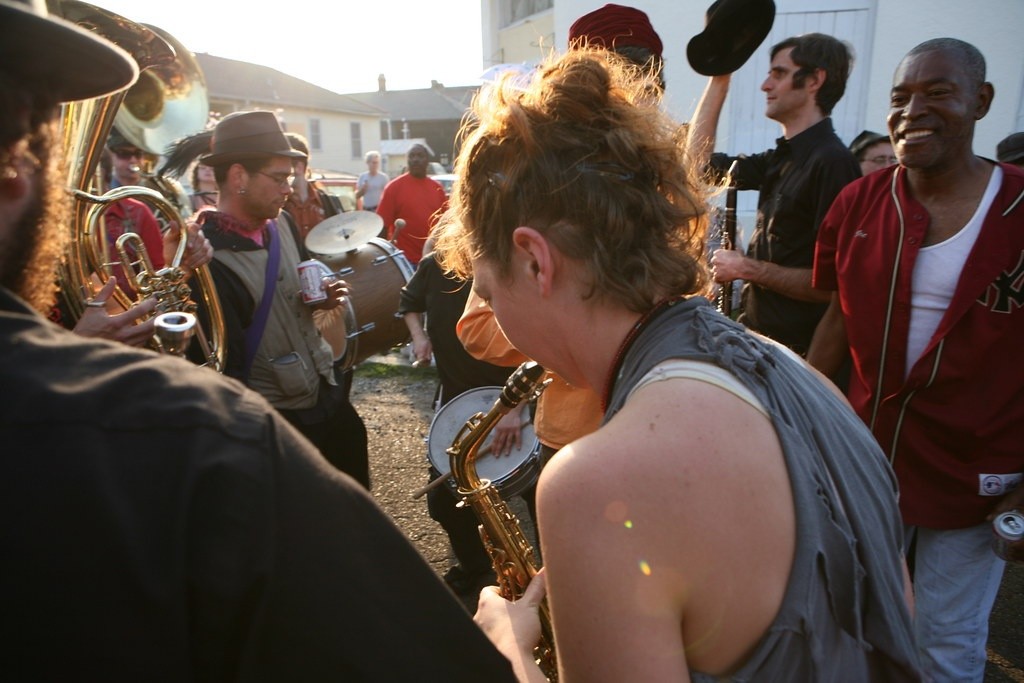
<box><xmin>198</xmin><ymin>111</ymin><xmax>307</xmax><ymax>166</ymax></box>
<box><xmin>1</xmin><ymin>2</ymin><xmax>139</xmax><ymax>104</ymax></box>
<box><xmin>849</xmin><ymin>131</ymin><xmax>889</xmax><ymax>156</ymax></box>
<box><xmin>285</xmin><ymin>132</ymin><xmax>309</xmax><ymax>162</ymax></box>
<box><xmin>686</xmin><ymin>0</ymin><xmax>776</xmax><ymax>76</ymax></box>
<box><xmin>997</xmin><ymin>132</ymin><xmax>1024</xmax><ymax>162</ymax></box>
<box><xmin>570</xmin><ymin>4</ymin><xmax>663</xmax><ymax>57</ymax></box>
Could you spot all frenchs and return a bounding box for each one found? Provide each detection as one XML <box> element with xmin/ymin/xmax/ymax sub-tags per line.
<box><xmin>47</xmin><ymin>1</ymin><xmax>227</xmax><ymax>379</ymax></box>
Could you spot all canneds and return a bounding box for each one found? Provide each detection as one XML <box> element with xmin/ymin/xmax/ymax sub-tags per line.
<box><xmin>296</xmin><ymin>260</ymin><xmax>328</xmax><ymax>304</ymax></box>
<box><xmin>991</xmin><ymin>511</ymin><xmax>1024</xmax><ymax>561</ymax></box>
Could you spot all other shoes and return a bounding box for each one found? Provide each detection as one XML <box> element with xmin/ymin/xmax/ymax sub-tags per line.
<box><xmin>444</xmin><ymin>565</ymin><xmax>484</xmax><ymax>595</ymax></box>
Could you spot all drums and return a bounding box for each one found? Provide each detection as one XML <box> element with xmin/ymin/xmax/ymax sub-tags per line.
<box><xmin>319</xmin><ymin>238</ymin><xmax>429</xmax><ymax>373</ymax></box>
<box><xmin>429</xmin><ymin>384</ymin><xmax>540</xmax><ymax>502</ymax></box>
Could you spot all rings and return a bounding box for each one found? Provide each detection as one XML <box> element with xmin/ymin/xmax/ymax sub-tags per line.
<box><xmin>82</xmin><ymin>298</ymin><xmax>107</xmax><ymax>307</ymax></box>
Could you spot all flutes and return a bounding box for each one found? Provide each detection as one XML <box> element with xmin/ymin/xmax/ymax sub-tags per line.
<box><xmin>716</xmin><ymin>161</ymin><xmax>741</xmax><ymax>322</ymax></box>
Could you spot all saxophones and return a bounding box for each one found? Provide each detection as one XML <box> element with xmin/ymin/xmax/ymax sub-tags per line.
<box><xmin>446</xmin><ymin>360</ymin><xmax>558</xmax><ymax>682</ymax></box>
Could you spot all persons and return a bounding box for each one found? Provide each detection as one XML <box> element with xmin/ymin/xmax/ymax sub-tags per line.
<box><xmin>0</xmin><ymin>0</ymin><xmax>521</xmax><ymax>683</ymax></box>
<box><xmin>997</xmin><ymin>130</ymin><xmax>1024</xmax><ymax>168</ymax></box>
<box><xmin>424</xmin><ymin>37</ymin><xmax>931</xmax><ymax>683</ymax></box>
<box><xmin>563</xmin><ymin>0</ymin><xmax>906</xmax><ymax>404</ymax></box>
<box><xmin>804</xmin><ymin>37</ymin><xmax>1024</xmax><ymax>683</ymax></box>
<box><xmin>51</xmin><ymin>109</ymin><xmax>447</xmax><ymax>494</ymax></box>
<box><xmin>403</xmin><ymin>207</ymin><xmax>548</xmax><ymax>579</ymax></box>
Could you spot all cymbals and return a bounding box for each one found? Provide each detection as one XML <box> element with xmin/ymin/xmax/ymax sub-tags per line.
<box><xmin>304</xmin><ymin>208</ymin><xmax>385</xmax><ymax>256</ymax></box>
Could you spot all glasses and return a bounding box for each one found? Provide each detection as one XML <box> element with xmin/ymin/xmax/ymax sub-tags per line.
<box><xmin>255</xmin><ymin>170</ymin><xmax>295</xmax><ymax>188</ymax></box>
<box><xmin>861</xmin><ymin>156</ymin><xmax>898</xmax><ymax>165</ymax></box>
<box><xmin>109</xmin><ymin>147</ymin><xmax>143</xmax><ymax>160</ymax></box>
